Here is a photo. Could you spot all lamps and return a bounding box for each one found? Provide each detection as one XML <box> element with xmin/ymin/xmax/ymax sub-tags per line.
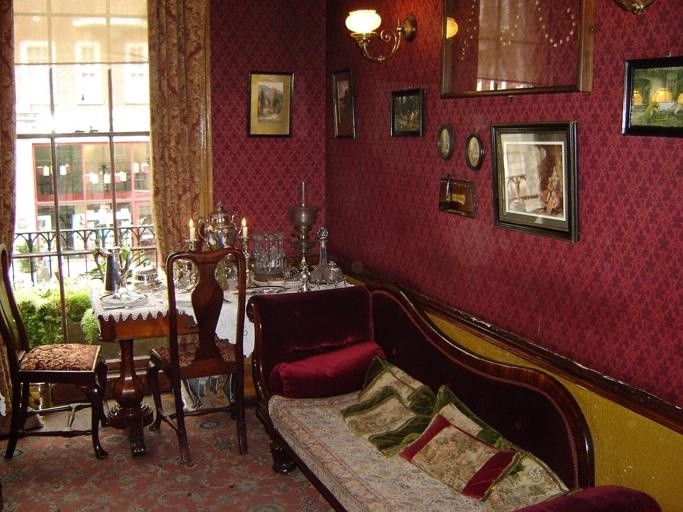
<box><xmin>345</xmin><ymin>10</ymin><xmax>417</xmax><ymax>64</ymax></box>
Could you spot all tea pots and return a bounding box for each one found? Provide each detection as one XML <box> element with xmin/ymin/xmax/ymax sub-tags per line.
<box><xmin>94</xmin><ymin>248</ymin><xmax>131</xmax><ymax>293</ymax></box>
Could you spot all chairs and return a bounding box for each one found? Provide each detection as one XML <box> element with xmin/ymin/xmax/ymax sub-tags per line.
<box><xmin>147</xmin><ymin>247</ymin><xmax>248</xmax><ymax>464</ymax></box>
<box><xmin>0</xmin><ymin>244</ymin><xmax>108</xmax><ymax>460</ymax></box>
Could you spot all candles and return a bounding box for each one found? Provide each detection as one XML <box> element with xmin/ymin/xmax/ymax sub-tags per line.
<box><xmin>189</xmin><ymin>219</ymin><xmax>195</xmax><ymax>240</ymax></box>
<box><xmin>241</xmin><ymin>218</ymin><xmax>247</xmax><ymax>237</ymax></box>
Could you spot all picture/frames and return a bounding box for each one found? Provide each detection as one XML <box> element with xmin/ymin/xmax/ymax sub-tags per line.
<box><xmin>247</xmin><ymin>69</ymin><xmax>295</xmax><ymax>137</ymax></box>
<box><xmin>436</xmin><ymin>124</ymin><xmax>454</xmax><ymax>161</ymax></box>
<box><xmin>489</xmin><ymin>119</ymin><xmax>580</xmax><ymax>243</ymax></box>
<box><xmin>331</xmin><ymin>69</ymin><xmax>357</xmax><ymax>140</ymax></box>
<box><xmin>439</xmin><ymin>0</ymin><xmax>593</xmax><ymax>99</ymax></box>
<box><xmin>621</xmin><ymin>56</ymin><xmax>683</xmax><ymax>138</ymax></box>
<box><xmin>464</xmin><ymin>133</ymin><xmax>485</xmax><ymax>171</ymax></box>
<box><xmin>390</xmin><ymin>88</ymin><xmax>424</xmax><ymax>138</ymax></box>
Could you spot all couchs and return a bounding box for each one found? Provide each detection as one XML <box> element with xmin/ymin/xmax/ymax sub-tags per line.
<box><xmin>246</xmin><ymin>280</ymin><xmax>683</xmax><ymax>512</ymax></box>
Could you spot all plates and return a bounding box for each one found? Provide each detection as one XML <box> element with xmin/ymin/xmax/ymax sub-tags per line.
<box><xmin>100</xmin><ymin>292</ymin><xmax>147</xmax><ymax>308</ymax></box>
